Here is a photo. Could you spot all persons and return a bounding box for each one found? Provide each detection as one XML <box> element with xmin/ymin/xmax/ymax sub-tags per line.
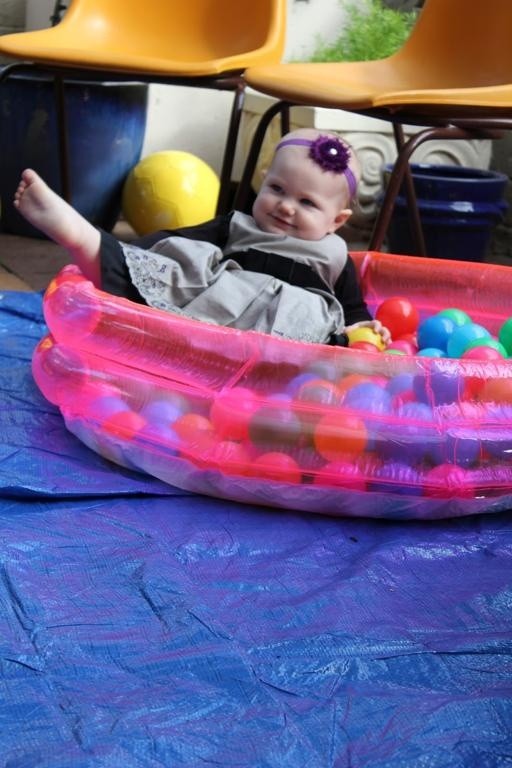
<box><xmin>11</xmin><ymin>124</ymin><xmax>392</xmax><ymax>389</ymax></box>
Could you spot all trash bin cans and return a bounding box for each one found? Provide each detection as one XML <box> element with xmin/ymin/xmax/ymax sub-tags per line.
<box><xmin>380</xmin><ymin>162</ymin><xmax>509</xmax><ymax>260</ymax></box>
<box><xmin>0</xmin><ymin>66</ymin><xmax>150</xmax><ymax>241</ymax></box>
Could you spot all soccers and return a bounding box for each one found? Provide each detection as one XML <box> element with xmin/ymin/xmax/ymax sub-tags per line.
<box><xmin>121</xmin><ymin>150</ymin><xmax>220</xmax><ymax>237</ymax></box>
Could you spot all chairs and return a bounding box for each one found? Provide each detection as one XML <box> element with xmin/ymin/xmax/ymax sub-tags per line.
<box><xmin>0</xmin><ymin>1</ymin><xmax>288</xmax><ymax>252</ymax></box>
<box><xmin>235</xmin><ymin>2</ymin><xmax>511</xmax><ymax>253</ymax></box>
